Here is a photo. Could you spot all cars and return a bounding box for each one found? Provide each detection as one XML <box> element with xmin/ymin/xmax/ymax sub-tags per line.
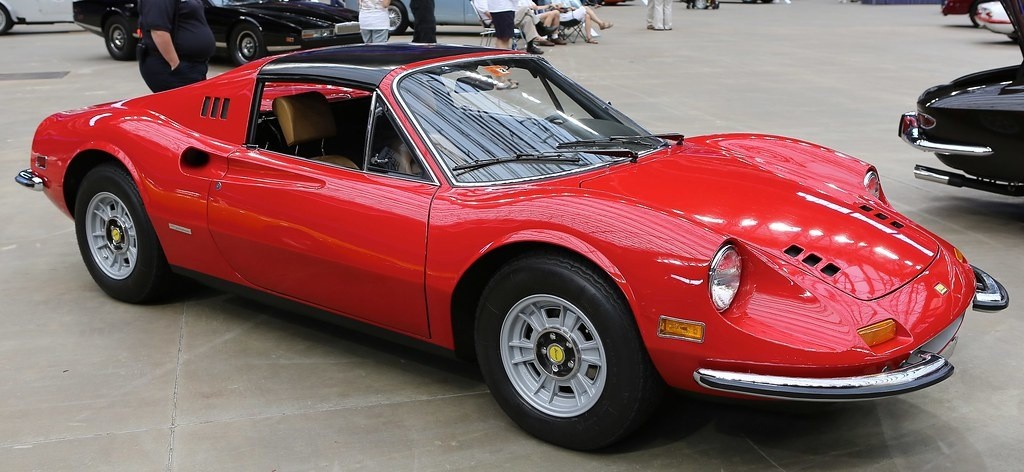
<box><xmin>0</xmin><ymin>0</ymin><xmax>79</xmax><ymax>36</ymax></box>
<box><xmin>344</xmin><ymin>0</ymin><xmax>635</xmax><ymax>36</ymax></box>
<box><xmin>940</xmin><ymin>0</ymin><xmax>1021</xmax><ymax>43</ymax></box>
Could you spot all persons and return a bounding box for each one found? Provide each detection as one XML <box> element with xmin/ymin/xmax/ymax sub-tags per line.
<box><xmin>644</xmin><ymin>0</ymin><xmax>720</xmax><ymax>31</ymax></box>
<box><xmin>358</xmin><ymin>0</ymin><xmax>391</xmax><ymax>43</ymax></box>
<box><xmin>473</xmin><ymin>0</ymin><xmax>614</xmax><ymax>89</ymax></box>
<box><xmin>139</xmin><ymin>0</ymin><xmax>216</xmax><ymax>94</ymax></box>
<box><xmin>409</xmin><ymin>0</ymin><xmax>437</xmax><ymax>43</ymax></box>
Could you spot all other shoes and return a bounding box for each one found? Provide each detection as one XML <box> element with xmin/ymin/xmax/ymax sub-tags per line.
<box><xmin>540</xmin><ymin>40</ymin><xmax>555</xmax><ymax>46</ymax></box>
<box><xmin>527</xmin><ymin>45</ymin><xmax>544</xmax><ymax>54</ymax></box>
<box><xmin>550</xmin><ymin>37</ymin><xmax>567</xmax><ymax>45</ymax></box>
<box><xmin>497</xmin><ymin>79</ymin><xmax>520</xmax><ymax>89</ymax></box>
<box><xmin>647</xmin><ymin>25</ymin><xmax>653</xmax><ymax>29</ymax></box>
<box><xmin>654</xmin><ymin>28</ymin><xmax>664</xmax><ymax>30</ymax></box>
<box><xmin>539</xmin><ymin>25</ymin><xmax>558</xmax><ymax>36</ymax></box>
<box><xmin>665</xmin><ymin>28</ymin><xmax>672</xmax><ymax>30</ymax></box>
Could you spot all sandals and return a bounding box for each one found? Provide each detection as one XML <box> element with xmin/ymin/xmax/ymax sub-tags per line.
<box><xmin>586</xmin><ymin>37</ymin><xmax>598</xmax><ymax>44</ymax></box>
<box><xmin>600</xmin><ymin>22</ymin><xmax>613</xmax><ymax>30</ymax></box>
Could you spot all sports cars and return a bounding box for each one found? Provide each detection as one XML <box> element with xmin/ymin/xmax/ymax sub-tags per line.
<box><xmin>72</xmin><ymin>0</ymin><xmax>391</xmax><ymax>69</ymax></box>
<box><xmin>895</xmin><ymin>0</ymin><xmax>1024</xmax><ymax>197</ymax></box>
<box><xmin>15</xmin><ymin>38</ymin><xmax>1011</xmax><ymax>455</ymax></box>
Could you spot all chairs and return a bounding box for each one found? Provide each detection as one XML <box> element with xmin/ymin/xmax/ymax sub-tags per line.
<box><xmin>470</xmin><ymin>1</ymin><xmax>526</xmax><ymax>50</ymax></box>
<box><xmin>558</xmin><ymin>22</ymin><xmax>587</xmax><ymax>44</ymax></box>
<box><xmin>395</xmin><ymin>75</ymin><xmax>465</xmax><ymax>178</ymax></box>
<box><xmin>272</xmin><ymin>92</ymin><xmax>360</xmax><ymax>172</ymax></box>
